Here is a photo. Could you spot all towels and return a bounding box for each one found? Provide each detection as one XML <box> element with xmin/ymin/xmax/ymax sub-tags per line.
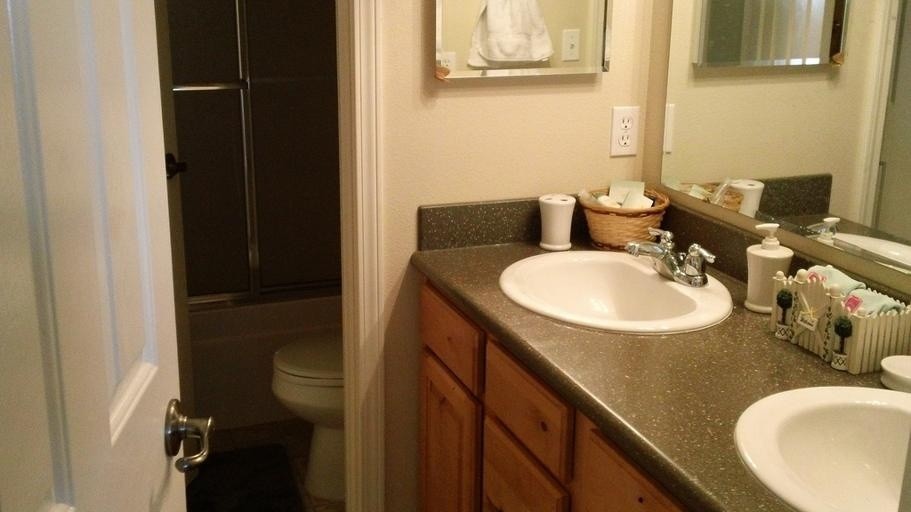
<box><xmin>466</xmin><ymin>0</ymin><xmax>555</xmax><ymax>70</ymax></box>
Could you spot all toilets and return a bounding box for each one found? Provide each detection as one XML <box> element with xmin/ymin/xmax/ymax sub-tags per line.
<box><xmin>269</xmin><ymin>331</ymin><xmax>347</xmax><ymax>503</ymax></box>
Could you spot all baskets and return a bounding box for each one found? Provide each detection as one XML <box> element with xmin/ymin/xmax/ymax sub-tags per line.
<box><xmin>675</xmin><ymin>181</ymin><xmax>744</xmax><ymax>214</ymax></box>
<box><xmin>576</xmin><ymin>183</ymin><xmax>672</xmax><ymax>253</ymax></box>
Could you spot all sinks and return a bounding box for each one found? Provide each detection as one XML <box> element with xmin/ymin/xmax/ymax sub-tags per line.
<box><xmin>497</xmin><ymin>250</ymin><xmax>734</xmax><ymax>335</ymax></box>
<box><xmin>836</xmin><ymin>232</ymin><xmax>911</xmax><ymax>277</ymax></box>
<box><xmin>732</xmin><ymin>386</ymin><xmax>911</xmax><ymax>512</ymax></box>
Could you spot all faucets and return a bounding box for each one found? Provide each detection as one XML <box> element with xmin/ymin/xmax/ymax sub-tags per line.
<box><xmin>625</xmin><ymin>240</ymin><xmax>685</xmax><ymax>279</ymax></box>
<box><xmin>803</xmin><ymin>222</ymin><xmax>841</xmax><ymax>238</ymax></box>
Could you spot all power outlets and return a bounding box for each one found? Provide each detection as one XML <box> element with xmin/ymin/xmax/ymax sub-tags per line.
<box><xmin>609</xmin><ymin>104</ymin><xmax>640</xmax><ymax>158</ymax></box>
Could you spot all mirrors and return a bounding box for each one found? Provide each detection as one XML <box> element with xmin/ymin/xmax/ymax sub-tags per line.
<box><xmin>644</xmin><ymin>1</ymin><xmax>910</xmax><ymax>295</ymax></box>
<box><xmin>434</xmin><ymin>0</ymin><xmax>612</xmax><ymax>83</ymax></box>
<box><xmin>690</xmin><ymin>0</ymin><xmax>851</xmax><ymax>83</ymax></box>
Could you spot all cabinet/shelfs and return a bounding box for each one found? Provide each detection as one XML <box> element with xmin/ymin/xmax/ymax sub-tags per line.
<box><xmin>482</xmin><ymin>337</ymin><xmax>573</xmax><ymax>511</ymax></box>
<box><xmin>579</xmin><ymin>426</ymin><xmax>682</xmax><ymax>509</ymax></box>
<box><xmin>412</xmin><ymin>291</ymin><xmax>485</xmax><ymax>510</ymax></box>
